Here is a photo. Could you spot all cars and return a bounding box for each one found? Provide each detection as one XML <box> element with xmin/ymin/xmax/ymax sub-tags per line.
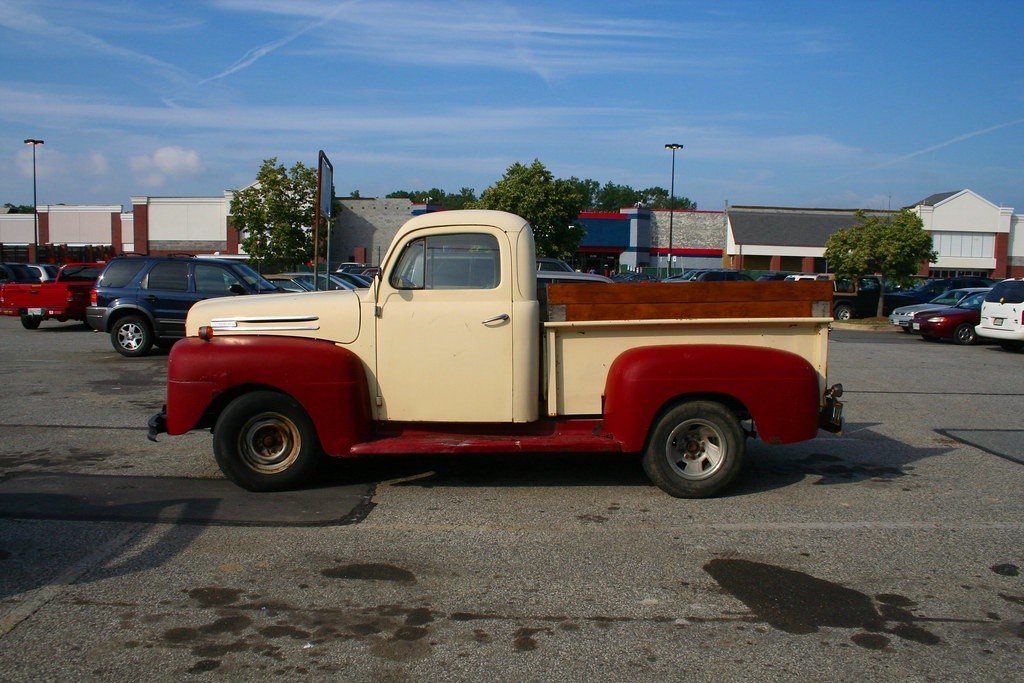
<box><xmin>662</xmin><ymin>268</ymin><xmax>741</xmax><ymax>283</ymax></box>
<box><xmin>610</xmin><ymin>271</ymin><xmax>662</xmax><ymax>284</ymax></box>
<box><xmin>336</xmin><ymin>262</ymin><xmax>380</xmax><ymax>279</ymax></box>
<box><xmin>234</xmin><ymin>272</ymin><xmax>373</xmax><ymax>295</ymax></box>
<box><xmin>907</xmin><ymin>289</ymin><xmax>1008</xmax><ymax>345</ymax></box>
<box><xmin>412</xmin><ymin>252</ymin><xmax>612</xmax><ymax>288</ymax></box>
<box><xmin>147</xmin><ymin>209</ymin><xmax>843</xmax><ymax>499</ymax></box>
<box><xmin>755</xmin><ymin>271</ymin><xmax>816</xmax><ymax>281</ymax></box>
<box><xmin>877</xmin><ymin>276</ymin><xmax>1004</xmax><ymax>313</ymax></box>
<box><xmin>888</xmin><ymin>287</ymin><xmax>995</xmax><ymax>334</ymax></box>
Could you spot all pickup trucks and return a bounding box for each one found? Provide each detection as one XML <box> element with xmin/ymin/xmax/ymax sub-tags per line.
<box><xmin>0</xmin><ymin>262</ymin><xmax>108</xmax><ymax>329</ymax></box>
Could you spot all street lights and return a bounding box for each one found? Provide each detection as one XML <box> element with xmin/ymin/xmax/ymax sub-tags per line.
<box><xmin>23</xmin><ymin>139</ymin><xmax>44</xmax><ymax>261</ymax></box>
<box><xmin>665</xmin><ymin>144</ymin><xmax>683</xmax><ymax>278</ymax></box>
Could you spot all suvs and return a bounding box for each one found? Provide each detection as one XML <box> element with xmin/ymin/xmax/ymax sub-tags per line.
<box><xmin>85</xmin><ymin>252</ymin><xmax>300</xmax><ymax>358</ymax></box>
<box><xmin>816</xmin><ymin>273</ymin><xmax>896</xmax><ymax>321</ymax></box>
<box><xmin>974</xmin><ymin>277</ymin><xmax>1024</xmax><ymax>350</ymax></box>
<box><xmin>0</xmin><ymin>263</ymin><xmax>59</xmax><ymax>285</ymax></box>
